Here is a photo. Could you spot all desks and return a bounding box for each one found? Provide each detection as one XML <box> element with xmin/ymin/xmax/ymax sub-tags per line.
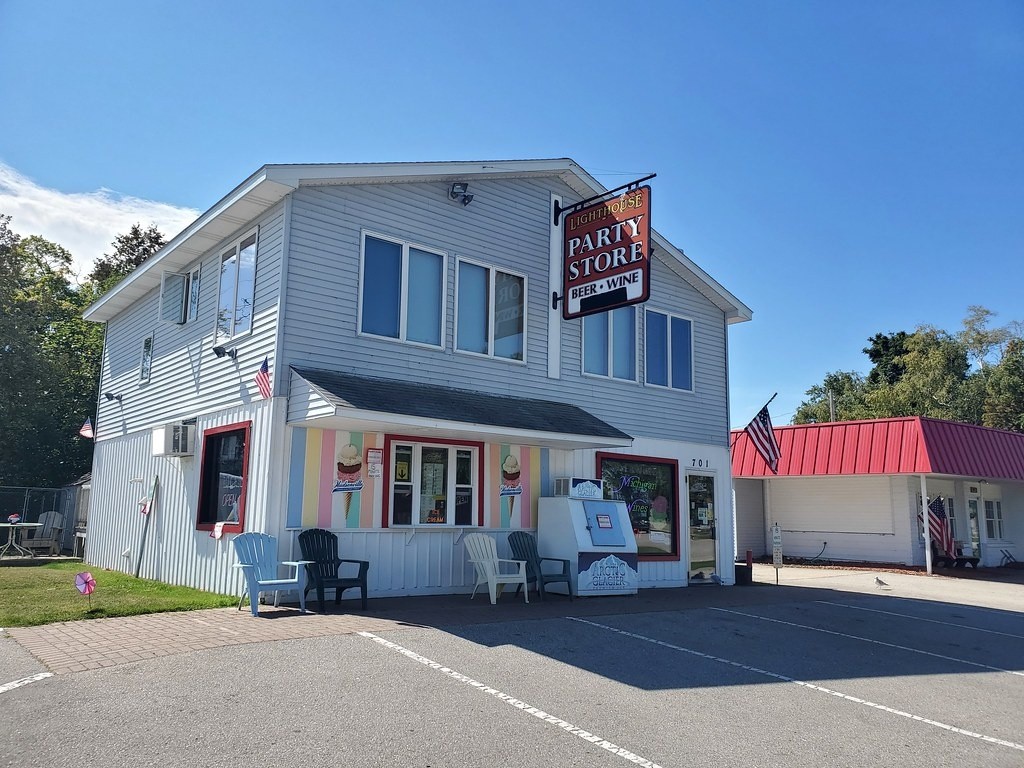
<box><xmin>0</xmin><ymin>523</ymin><xmax>44</xmax><ymax>559</ymax></box>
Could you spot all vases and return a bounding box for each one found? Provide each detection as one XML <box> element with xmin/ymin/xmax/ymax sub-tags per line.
<box><xmin>10</xmin><ymin>520</ymin><xmax>17</xmax><ymax>525</ymax></box>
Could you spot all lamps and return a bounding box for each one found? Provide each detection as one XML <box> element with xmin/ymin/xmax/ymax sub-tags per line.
<box><xmin>213</xmin><ymin>347</ymin><xmax>237</xmax><ymax>360</ymax></box>
<box><xmin>450</xmin><ymin>182</ymin><xmax>475</xmax><ymax>207</ymax></box>
<box><xmin>105</xmin><ymin>392</ymin><xmax>122</xmax><ymax>402</ymax></box>
<box><xmin>978</xmin><ymin>479</ymin><xmax>989</xmax><ymax>485</ymax></box>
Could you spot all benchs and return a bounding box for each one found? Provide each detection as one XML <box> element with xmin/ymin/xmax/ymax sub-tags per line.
<box><xmin>931</xmin><ymin>540</ymin><xmax>979</xmax><ymax>568</ymax></box>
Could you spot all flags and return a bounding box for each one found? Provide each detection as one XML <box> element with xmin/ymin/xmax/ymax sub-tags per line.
<box><xmin>253</xmin><ymin>358</ymin><xmax>271</xmax><ymax>398</ymax></box>
<box><xmin>744</xmin><ymin>405</ymin><xmax>782</xmax><ymax>475</ymax></box>
<box><xmin>918</xmin><ymin>496</ymin><xmax>953</xmax><ymax>557</ymax></box>
<box><xmin>79</xmin><ymin>418</ymin><xmax>93</xmax><ymax>438</ymax></box>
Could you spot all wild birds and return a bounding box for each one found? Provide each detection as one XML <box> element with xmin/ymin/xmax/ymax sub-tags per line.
<box><xmin>874</xmin><ymin>577</ymin><xmax>888</xmax><ymax>589</ymax></box>
<box><xmin>709</xmin><ymin>573</ymin><xmax>725</xmax><ymax>585</ymax></box>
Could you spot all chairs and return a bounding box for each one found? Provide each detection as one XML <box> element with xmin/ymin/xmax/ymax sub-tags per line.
<box><xmin>464</xmin><ymin>532</ymin><xmax>529</xmax><ymax>604</ymax></box>
<box><xmin>21</xmin><ymin>510</ymin><xmax>64</xmax><ymax>557</ymax></box>
<box><xmin>294</xmin><ymin>529</ymin><xmax>369</xmax><ymax>613</ymax></box>
<box><xmin>508</xmin><ymin>530</ymin><xmax>573</xmax><ymax>602</ymax></box>
<box><xmin>232</xmin><ymin>532</ymin><xmax>307</xmax><ymax>617</ymax></box>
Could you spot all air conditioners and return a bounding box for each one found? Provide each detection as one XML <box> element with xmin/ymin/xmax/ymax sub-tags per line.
<box><xmin>151</xmin><ymin>424</ymin><xmax>194</xmax><ymax>457</ymax></box>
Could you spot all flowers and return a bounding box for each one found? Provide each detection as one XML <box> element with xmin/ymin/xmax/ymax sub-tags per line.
<box><xmin>7</xmin><ymin>514</ymin><xmax>20</xmax><ymax>522</ymax></box>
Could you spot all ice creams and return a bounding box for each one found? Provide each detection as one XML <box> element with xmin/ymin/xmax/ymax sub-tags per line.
<box><xmin>502</xmin><ymin>454</ymin><xmax>521</xmax><ymax>516</ymax></box>
<box><xmin>337</xmin><ymin>443</ymin><xmax>362</xmax><ymax>519</ymax></box>
